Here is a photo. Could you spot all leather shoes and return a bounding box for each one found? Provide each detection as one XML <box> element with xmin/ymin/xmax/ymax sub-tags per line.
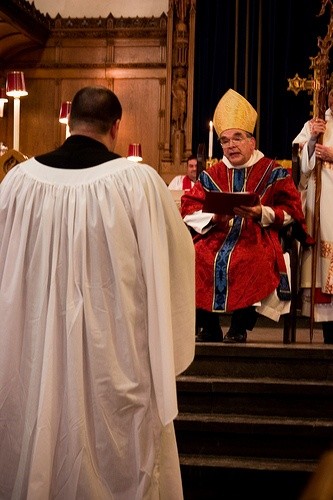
<box><xmin>223</xmin><ymin>328</ymin><xmax>247</xmax><ymax>343</ymax></box>
<box><xmin>195</xmin><ymin>327</ymin><xmax>223</xmax><ymax>342</ymax></box>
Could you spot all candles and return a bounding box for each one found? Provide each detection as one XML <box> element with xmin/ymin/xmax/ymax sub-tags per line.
<box><xmin>208</xmin><ymin>121</ymin><xmax>214</xmax><ymax>157</ymax></box>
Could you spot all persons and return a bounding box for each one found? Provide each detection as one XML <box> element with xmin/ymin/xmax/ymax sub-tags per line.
<box><xmin>0</xmin><ymin>83</ymin><xmax>196</xmax><ymax>500</ymax></box>
<box><xmin>169</xmin><ymin>89</ymin><xmax>333</xmax><ymax>344</ymax></box>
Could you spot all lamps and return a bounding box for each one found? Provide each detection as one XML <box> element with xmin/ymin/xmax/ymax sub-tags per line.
<box><xmin>127</xmin><ymin>144</ymin><xmax>143</xmax><ymax>163</ymax></box>
<box><xmin>6</xmin><ymin>71</ymin><xmax>28</xmax><ymax>152</ymax></box>
<box><xmin>0</xmin><ymin>88</ymin><xmax>8</xmax><ymax>117</ymax></box>
<box><xmin>59</xmin><ymin>101</ymin><xmax>72</xmax><ymax>139</ymax></box>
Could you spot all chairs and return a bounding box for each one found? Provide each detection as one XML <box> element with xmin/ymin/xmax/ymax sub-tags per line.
<box><xmin>191</xmin><ymin>144</ymin><xmax>304</xmax><ymax>343</ymax></box>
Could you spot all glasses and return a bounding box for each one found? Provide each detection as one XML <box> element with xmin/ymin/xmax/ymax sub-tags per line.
<box><xmin>220</xmin><ymin>136</ymin><xmax>252</xmax><ymax>147</ymax></box>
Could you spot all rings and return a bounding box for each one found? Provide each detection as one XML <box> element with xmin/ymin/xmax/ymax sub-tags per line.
<box><xmin>319</xmin><ymin>152</ymin><xmax>321</xmax><ymax>155</ymax></box>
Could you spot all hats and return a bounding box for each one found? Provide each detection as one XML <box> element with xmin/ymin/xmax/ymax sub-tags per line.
<box><xmin>213</xmin><ymin>88</ymin><xmax>258</xmax><ymax>138</ymax></box>
